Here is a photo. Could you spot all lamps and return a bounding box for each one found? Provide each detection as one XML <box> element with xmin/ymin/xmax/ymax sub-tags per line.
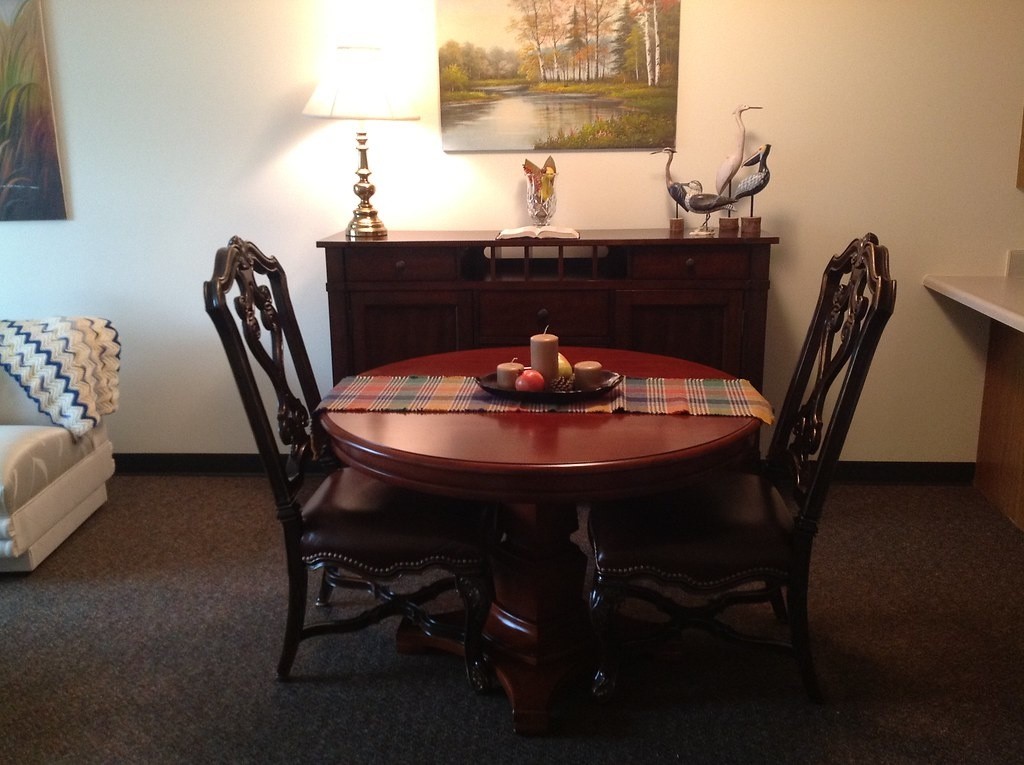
<box><xmin>301</xmin><ymin>46</ymin><xmax>422</xmax><ymax>237</ymax></box>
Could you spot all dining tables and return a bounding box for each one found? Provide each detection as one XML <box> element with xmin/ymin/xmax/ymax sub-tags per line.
<box><xmin>315</xmin><ymin>346</ymin><xmax>776</xmax><ymax>736</ymax></box>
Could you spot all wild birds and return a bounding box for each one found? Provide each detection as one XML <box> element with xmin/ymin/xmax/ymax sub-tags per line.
<box><xmin>715</xmin><ymin>104</ymin><xmax>762</xmax><ymax>195</ymax></box>
<box><xmin>680</xmin><ymin>180</ymin><xmax>739</xmax><ymax>233</ymax></box>
<box><xmin>650</xmin><ymin>147</ymin><xmax>690</xmax><ymax>212</ymax></box>
<box><xmin>733</xmin><ymin>143</ymin><xmax>773</xmax><ymax>200</ymax></box>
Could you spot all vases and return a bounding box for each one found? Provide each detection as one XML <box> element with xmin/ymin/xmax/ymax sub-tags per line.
<box><xmin>525</xmin><ymin>173</ymin><xmax>558</xmax><ymax>226</ymax></box>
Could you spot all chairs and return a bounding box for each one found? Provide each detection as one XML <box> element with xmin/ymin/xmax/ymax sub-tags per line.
<box><xmin>584</xmin><ymin>232</ymin><xmax>898</xmax><ymax>710</ymax></box>
<box><xmin>201</xmin><ymin>235</ymin><xmax>512</xmax><ymax>697</ymax></box>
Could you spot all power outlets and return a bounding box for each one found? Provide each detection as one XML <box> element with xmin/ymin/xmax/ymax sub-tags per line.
<box><xmin>1005</xmin><ymin>249</ymin><xmax>1024</xmax><ymax>279</ymax></box>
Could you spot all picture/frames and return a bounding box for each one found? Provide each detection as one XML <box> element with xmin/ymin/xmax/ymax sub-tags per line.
<box><xmin>0</xmin><ymin>1</ymin><xmax>70</xmax><ymax>223</ymax></box>
<box><xmin>435</xmin><ymin>1</ymin><xmax>683</xmax><ymax>155</ymax></box>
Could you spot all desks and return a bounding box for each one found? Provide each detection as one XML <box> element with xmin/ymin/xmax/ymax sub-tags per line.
<box><xmin>923</xmin><ymin>274</ymin><xmax>1023</xmax><ymax>534</ymax></box>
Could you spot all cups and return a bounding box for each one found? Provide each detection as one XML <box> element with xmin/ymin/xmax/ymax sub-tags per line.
<box><xmin>526</xmin><ymin>171</ymin><xmax>557</xmax><ymax>229</ymax></box>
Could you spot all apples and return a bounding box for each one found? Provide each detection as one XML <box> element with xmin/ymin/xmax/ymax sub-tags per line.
<box><xmin>515</xmin><ymin>369</ymin><xmax>545</xmax><ymax>391</ymax></box>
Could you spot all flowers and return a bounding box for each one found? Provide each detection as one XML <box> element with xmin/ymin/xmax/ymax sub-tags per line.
<box><xmin>522</xmin><ymin>156</ymin><xmax>556</xmax><ymax>200</ymax></box>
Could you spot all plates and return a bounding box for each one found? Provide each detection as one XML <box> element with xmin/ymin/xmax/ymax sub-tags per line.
<box><xmin>474</xmin><ymin>367</ymin><xmax>624</xmax><ymax>403</ymax></box>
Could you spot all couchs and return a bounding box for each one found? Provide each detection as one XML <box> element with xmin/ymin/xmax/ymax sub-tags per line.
<box><xmin>0</xmin><ymin>317</ymin><xmax>122</xmax><ymax>573</ymax></box>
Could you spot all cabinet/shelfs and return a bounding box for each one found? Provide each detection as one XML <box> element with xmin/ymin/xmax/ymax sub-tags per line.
<box><xmin>314</xmin><ymin>226</ymin><xmax>780</xmax><ymax>393</ymax></box>
